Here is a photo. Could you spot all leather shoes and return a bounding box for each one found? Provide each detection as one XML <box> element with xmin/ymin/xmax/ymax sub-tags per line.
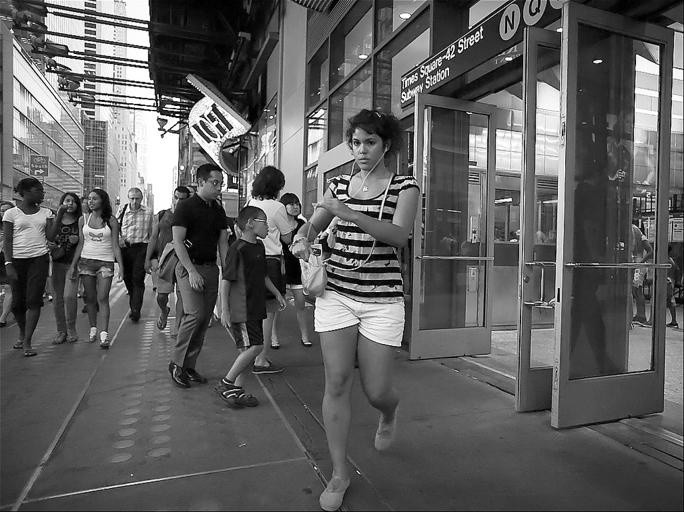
<box><xmin>301</xmin><ymin>337</ymin><xmax>312</xmax><ymax>347</ymax></box>
<box><xmin>270</xmin><ymin>336</ymin><xmax>280</xmax><ymax>350</ymax></box>
<box><xmin>168</xmin><ymin>360</ymin><xmax>192</xmax><ymax>388</ymax></box>
<box><xmin>185</xmin><ymin>367</ymin><xmax>207</xmax><ymax>385</ymax></box>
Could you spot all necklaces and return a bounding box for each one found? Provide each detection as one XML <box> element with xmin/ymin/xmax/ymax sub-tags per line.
<box><xmin>360</xmin><ymin>172</ymin><xmax>370</xmax><ymax>194</ymax></box>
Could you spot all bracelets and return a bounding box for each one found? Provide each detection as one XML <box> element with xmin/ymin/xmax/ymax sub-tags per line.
<box><xmin>4</xmin><ymin>262</ymin><xmax>12</xmax><ymax>265</ymax></box>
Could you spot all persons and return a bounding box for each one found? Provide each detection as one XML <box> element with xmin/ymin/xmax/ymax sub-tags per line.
<box><xmin>1</xmin><ymin>177</ymin><xmax>68</xmax><ymax>357</ymax></box>
<box><xmin>48</xmin><ymin>191</ymin><xmax>84</xmax><ymax>344</ymax></box>
<box><xmin>168</xmin><ymin>164</ymin><xmax>312</xmax><ymax>388</ymax></box>
<box><xmin>290</xmin><ymin>108</ymin><xmax>421</xmax><ymax>512</ymax></box>
<box><xmin>116</xmin><ymin>188</ymin><xmax>154</xmax><ymax>322</ymax></box>
<box><xmin>217</xmin><ymin>206</ymin><xmax>287</xmax><ymax>411</ymax></box>
<box><xmin>76</xmin><ymin>189</ymin><xmax>125</xmax><ymax>347</ymax></box>
<box><xmin>144</xmin><ymin>186</ymin><xmax>193</xmax><ymax>339</ymax></box>
<box><xmin>643</xmin><ymin>244</ymin><xmax>679</xmax><ymax>328</ymax></box>
<box><xmin>632</xmin><ymin>223</ymin><xmax>653</xmax><ymax>328</ymax></box>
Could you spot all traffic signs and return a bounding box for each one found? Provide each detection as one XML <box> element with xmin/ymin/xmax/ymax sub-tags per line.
<box><xmin>30</xmin><ymin>154</ymin><xmax>49</xmax><ymax>177</ymax></box>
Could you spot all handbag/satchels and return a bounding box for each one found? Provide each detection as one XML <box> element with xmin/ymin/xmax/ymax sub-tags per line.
<box><xmin>156</xmin><ymin>239</ymin><xmax>180</xmax><ymax>283</ymax></box>
<box><xmin>298</xmin><ymin>245</ymin><xmax>329</xmax><ymax>298</ymax></box>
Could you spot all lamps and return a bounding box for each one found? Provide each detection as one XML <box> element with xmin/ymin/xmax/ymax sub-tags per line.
<box><xmin>12</xmin><ymin>0</ymin><xmax>191</xmax><ymax>137</ymax></box>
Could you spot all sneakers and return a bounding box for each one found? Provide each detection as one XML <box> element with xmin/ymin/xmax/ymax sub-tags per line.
<box><xmin>374</xmin><ymin>405</ymin><xmax>399</xmax><ymax>453</ymax></box>
<box><xmin>216</xmin><ymin>359</ymin><xmax>286</xmax><ymax>407</ymax></box>
<box><xmin>55</xmin><ymin>327</ymin><xmax>109</xmax><ymax>348</ymax></box>
<box><xmin>318</xmin><ymin>471</ymin><xmax>351</xmax><ymax>512</ymax></box>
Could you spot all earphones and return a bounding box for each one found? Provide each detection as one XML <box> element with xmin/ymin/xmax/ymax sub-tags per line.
<box><xmin>384</xmin><ymin>143</ymin><xmax>389</xmax><ymax>152</ymax></box>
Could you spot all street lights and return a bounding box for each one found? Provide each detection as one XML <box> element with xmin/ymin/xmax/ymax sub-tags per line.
<box><xmin>155</xmin><ymin>115</ymin><xmax>193</xmax><ymax>186</ymax></box>
<box><xmin>86</xmin><ymin>144</ymin><xmax>122</xmax><ymax>208</ymax></box>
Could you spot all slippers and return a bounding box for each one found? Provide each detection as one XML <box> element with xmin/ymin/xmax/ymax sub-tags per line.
<box><xmin>24</xmin><ymin>349</ymin><xmax>33</xmax><ymax>356</ymax></box>
<box><xmin>14</xmin><ymin>340</ymin><xmax>23</xmax><ymax>348</ymax></box>
<box><xmin>156</xmin><ymin>306</ymin><xmax>171</xmax><ymax>330</ymax></box>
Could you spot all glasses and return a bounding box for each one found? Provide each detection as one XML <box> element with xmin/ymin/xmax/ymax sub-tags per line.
<box><xmin>253</xmin><ymin>218</ymin><xmax>267</xmax><ymax>224</ymax></box>
<box><xmin>206</xmin><ymin>180</ymin><xmax>226</xmax><ymax>188</ymax></box>
